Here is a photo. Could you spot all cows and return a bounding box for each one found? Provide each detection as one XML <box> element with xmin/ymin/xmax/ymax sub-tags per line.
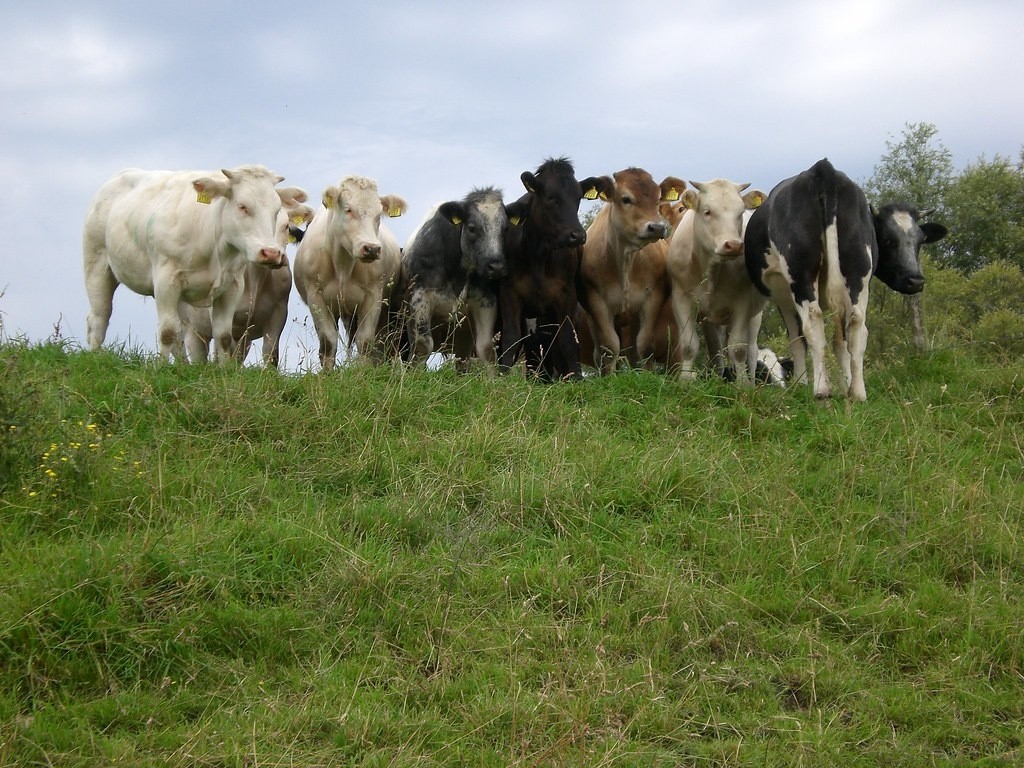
<box><xmin>498</xmin><ymin>154</ymin><xmax>606</xmax><ymax>382</ymax></box>
<box><xmin>293</xmin><ymin>176</ymin><xmax>410</xmax><ymax>372</ymax></box>
<box><xmin>83</xmin><ymin>164</ymin><xmax>318</xmax><ymax>373</ymax></box>
<box><xmin>742</xmin><ymin>157</ymin><xmax>950</xmax><ymax>405</ymax></box>
<box><xmin>658</xmin><ymin>179</ymin><xmax>769</xmax><ymax>387</ymax></box>
<box><xmin>578</xmin><ymin>166</ymin><xmax>687</xmax><ymax>380</ymax></box>
<box><xmin>386</xmin><ymin>186</ymin><xmax>528</xmax><ymax>379</ymax></box>
